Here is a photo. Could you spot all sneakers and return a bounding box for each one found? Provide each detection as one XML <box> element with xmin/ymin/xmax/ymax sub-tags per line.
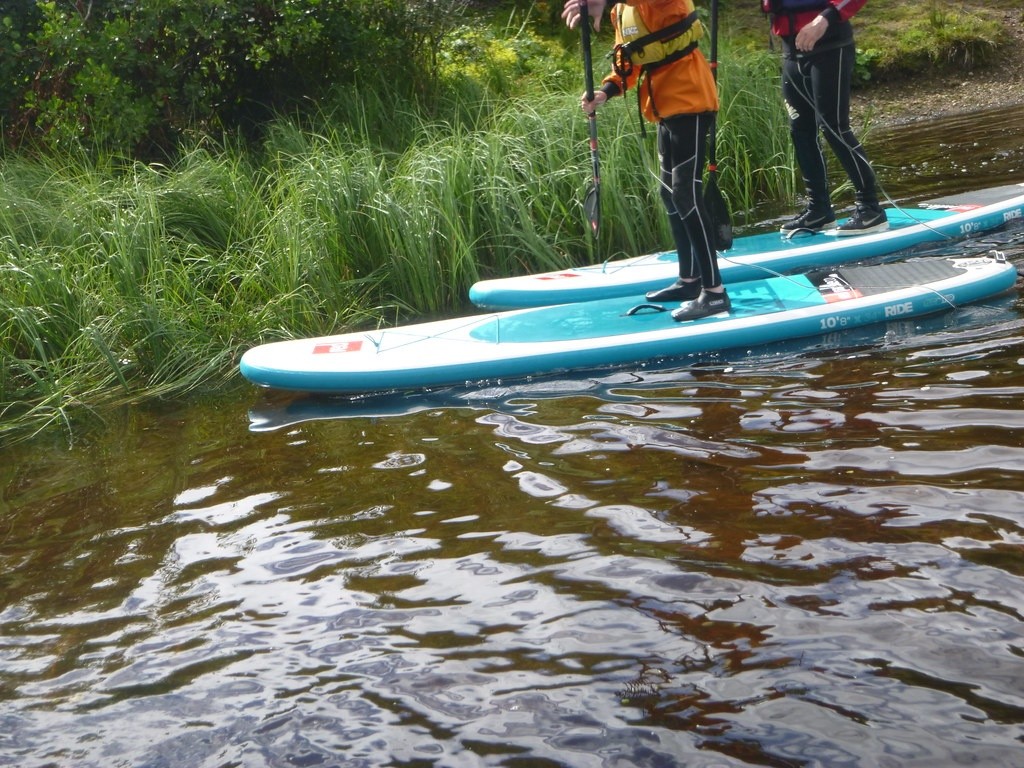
<box><xmin>836</xmin><ymin>201</ymin><xmax>889</xmax><ymax>236</ymax></box>
<box><xmin>780</xmin><ymin>210</ymin><xmax>837</xmax><ymax>234</ymax></box>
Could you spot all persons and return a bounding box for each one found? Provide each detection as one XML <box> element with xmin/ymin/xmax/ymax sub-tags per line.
<box><xmin>761</xmin><ymin>0</ymin><xmax>889</xmax><ymax>236</ymax></box>
<box><xmin>561</xmin><ymin>0</ymin><xmax>732</xmax><ymax>320</ymax></box>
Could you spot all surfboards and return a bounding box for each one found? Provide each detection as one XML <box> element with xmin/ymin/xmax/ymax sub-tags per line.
<box><xmin>465</xmin><ymin>176</ymin><xmax>1024</xmax><ymax>309</ymax></box>
<box><xmin>230</xmin><ymin>247</ymin><xmax>1016</xmax><ymax>395</ymax></box>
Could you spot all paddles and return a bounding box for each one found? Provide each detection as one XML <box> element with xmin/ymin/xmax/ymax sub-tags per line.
<box><xmin>702</xmin><ymin>0</ymin><xmax>734</xmax><ymax>250</ymax></box>
<box><xmin>574</xmin><ymin>4</ymin><xmax>605</xmax><ymax>238</ymax></box>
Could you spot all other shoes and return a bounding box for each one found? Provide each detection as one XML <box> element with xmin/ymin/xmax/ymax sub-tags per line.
<box><xmin>646</xmin><ymin>277</ymin><xmax>702</xmax><ymax>301</ymax></box>
<box><xmin>670</xmin><ymin>287</ymin><xmax>731</xmax><ymax>321</ymax></box>
